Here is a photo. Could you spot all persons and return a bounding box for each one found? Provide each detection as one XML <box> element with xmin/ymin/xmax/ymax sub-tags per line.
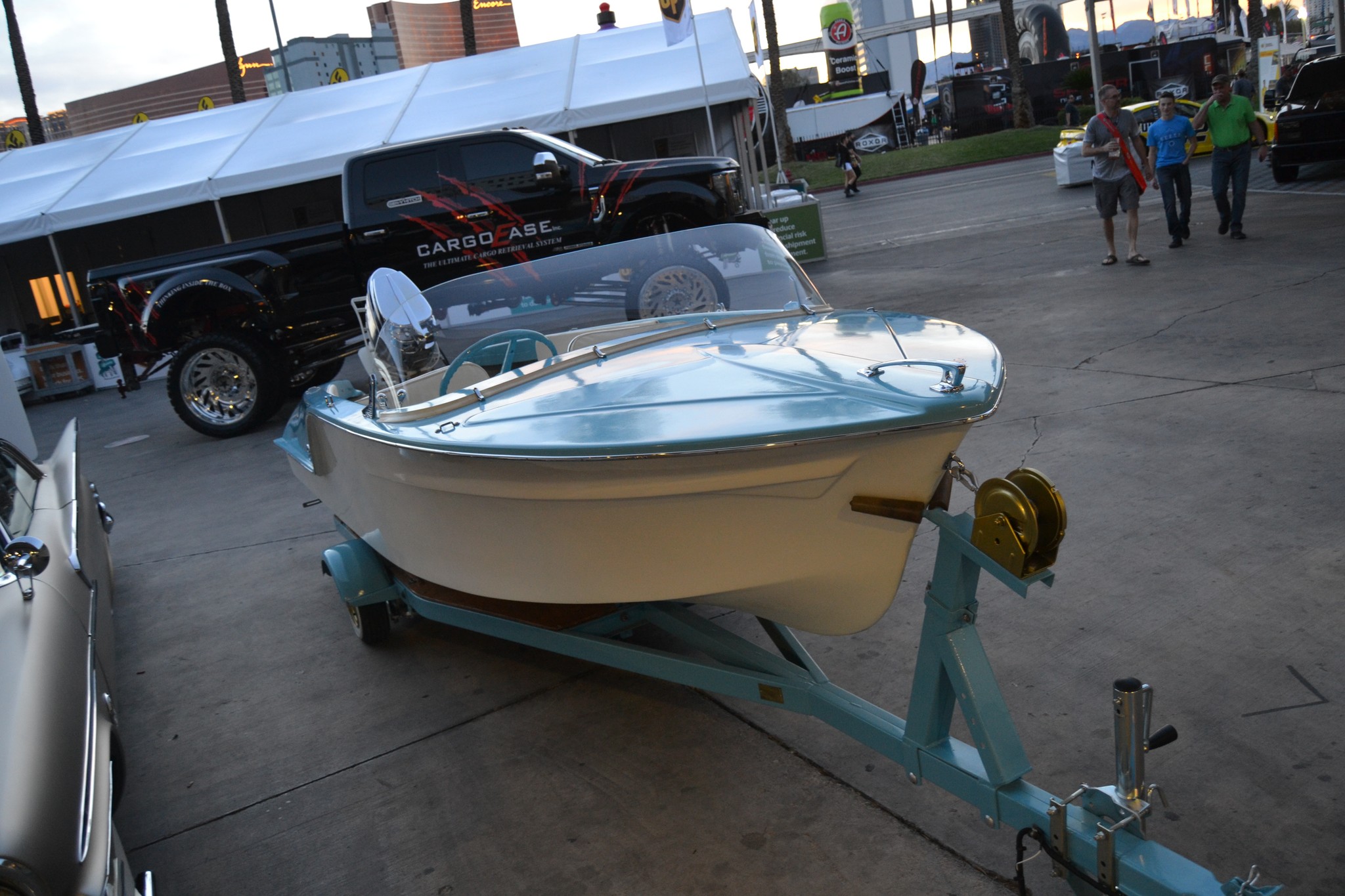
<box><xmin>1261</xmin><ymin>80</ymin><xmax>1267</xmax><ymax>113</ymax></box>
<box><xmin>837</xmin><ymin>134</ymin><xmax>857</xmax><ymax>198</ymax></box>
<box><xmin>843</xmin><ymin>130</ymin><xmax>863</xmax><ymax>193</ymax></box>
<box><xmin>1146</xmin><ymin>91</ymin><xmax>1198</xmax><ymax>248</ymax></box>
<box><xmin>1065</xmin><ymin>94</ymin><xmax>1082</xmax><ymax>128</ymax></box>
<box><xmin>1081</xmin><ymin>84</ymin><xmax>1153</xmax><ymax>267</ymax></box>
<box><xmin>1190</xmin><ymin>73</ymin><xmax>1269</xmax><ymax>240</ymax></box>
<box><xmin>1274</xmin><ymin>64</ymin><xmax>1296</xmax><ymax>116</ymax></box>
<box><xmin>1231</xmin><ymin>69</ymin><xmax>1258</xmax><ymax>112</ymax></box>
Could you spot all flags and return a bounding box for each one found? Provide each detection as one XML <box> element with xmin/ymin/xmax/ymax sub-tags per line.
<box><xmin>1197</xmin><ymin>0</ymin><xmax>1200</xmax><ymax>18</ymax></box>
<box><xmin>1185</xmin><ymin>0</ymin><xmax>1191</xmax><ymax>17</ymax></box>
<box><xmin>1147</xmin><ymin>0</ymin><xmax>1155</xmax><ymax>21</ymax></box>
<box><xmin>1173</xmin><ymin>0</ymin><xmax>1178</xmax><ymax>16</ymax></box>
<box><xmin>658</xmin><ymin>0</ymin><xmax>694</xmax><ymax>47</ymax></box>
<box><xmin>1042</xmin><ymin>17</ymin><xmax>1048</xmax><ymax>56</ymax></box>
<box><xmin>748</xmin><ymin>0</ymin><xmax>765</xmax><ymax>69</ymax></box>
<box><xmin>1110</xmin><ymin>0</ymin><xmax>1117</xmax><ymax>34</ymax></box>
<box><xmin>1212</xmin><ymin>1</ymin><xmax>1312</xmax><ymax>49</ymax></box>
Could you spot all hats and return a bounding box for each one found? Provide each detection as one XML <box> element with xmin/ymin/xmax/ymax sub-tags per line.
<box><xmin>1211</xmin><ymin>74</ymin><xmax>1230</xmax><ymax>85</ymax></box>
<box><xmin>1234</xmin><ymin>68</ymin><xmax>1247</xmax><ymax>76</ymax></box>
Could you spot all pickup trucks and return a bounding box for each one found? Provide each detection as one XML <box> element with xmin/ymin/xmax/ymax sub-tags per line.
<box><xmin>86</xmin><ymin>127</ymin><xmax>770</xmax><ymax>437</ymax></box>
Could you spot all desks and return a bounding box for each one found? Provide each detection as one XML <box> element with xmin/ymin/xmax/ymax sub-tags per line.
<box><xmin>19</xmin><ymin>343</ymin><xmax>96</xmax><ymax>399</ymax></box>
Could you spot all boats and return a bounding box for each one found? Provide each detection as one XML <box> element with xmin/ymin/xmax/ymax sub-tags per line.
<box><xmin>272</xmin><ymin>223</ymin><xmax>1004</xmax><ymax>635</ymax></box>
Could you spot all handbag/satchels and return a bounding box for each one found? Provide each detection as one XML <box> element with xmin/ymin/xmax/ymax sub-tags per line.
<box><xmin>1124</xmin><ymin>152</ymin><xmax>1147</xmax><ymax>195</ymax></box>
<box><xmin>835</xmin><ymin>153</ymin><xmax>842</xmax><ymax>168</ymax></box>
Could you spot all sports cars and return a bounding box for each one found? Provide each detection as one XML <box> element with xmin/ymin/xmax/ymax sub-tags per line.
<box><xmin>0</xmin><ymin>420</ymin><xmax>158</xmax><ymax>896</ymax></box>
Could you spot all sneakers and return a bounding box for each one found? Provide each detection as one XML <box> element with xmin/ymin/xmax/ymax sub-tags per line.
<box><xmin>1180</xmin><ymin>225</ymin><xmax>1190</xmax><ymax>239</ymax></box>
<box><xmin>1230</xmin><ymin>223</ymin><xmax>1246</xmax><ymax>239</ymax></box>
<box><xmin>1218</xmin><ymin>214</ymin><xmax>1232</xmax><ymax>234</ymax></box>
<box><xmin>1168</xmin><ymin>238</ymin><xmax>1182</xmax><ymax>248</ymax></box>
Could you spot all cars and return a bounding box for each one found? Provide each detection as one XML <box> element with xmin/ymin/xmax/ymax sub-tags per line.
<box><xmin>1263</xmin><ymin>34</ymin><xmax>1345</xmax><ymax>184</ymax></box>
<box><xmin>1057</xmin><ymin>99</ymin><xmax>1274</xmax><ymax>162</ymax></box>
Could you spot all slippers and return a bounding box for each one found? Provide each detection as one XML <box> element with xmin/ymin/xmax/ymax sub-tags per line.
<box><xmin>1126</xmin><ymin>254</ymin><xmax>1150</xmax><ymax>264</ymax></box>
<box><xmin>1102</xmin><ymin>255</ymin><xmax>1117</xmax><ymax>265</ymax></box>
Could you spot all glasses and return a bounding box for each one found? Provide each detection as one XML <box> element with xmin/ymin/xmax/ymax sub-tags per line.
<box><xmin>1105</xmin><ymin>93</ymin><xmax>1121</xmax><ymax>100</ymax></box>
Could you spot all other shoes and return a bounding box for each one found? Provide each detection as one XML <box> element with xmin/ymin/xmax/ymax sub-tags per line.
<box><xmin>853</xmin><ymin>189</ymin><xmax>860</xmax><ymax>192</ymax></box>
<box><xmin>844</xmin><ymin>190</ymin><xmax>853</xmax><ymax>197</ymax></box>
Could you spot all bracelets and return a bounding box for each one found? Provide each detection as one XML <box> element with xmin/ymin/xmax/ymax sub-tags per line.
<box><xmin>1259</xmin><ymin>142</ymin><xmax>1267</xmax><ymax>147</ymax></box>
